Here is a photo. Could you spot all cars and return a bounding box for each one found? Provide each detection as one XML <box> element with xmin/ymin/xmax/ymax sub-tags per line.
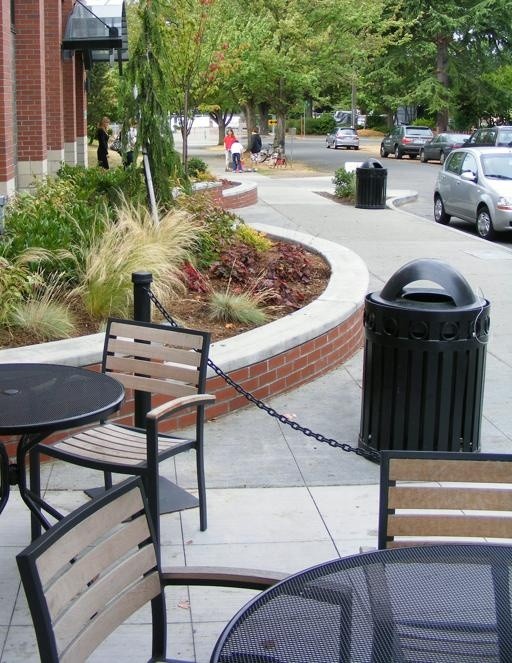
<box><xmin>325</xmin><ymin>127</ymin><xmax>360</xmax><ymax>150</ymax></box>
<box><xmin>419</xmin><ymin>133</ymin><xmax>475</xmax><ymax>164</ymax></box>
<box><xmin>433</xmin><ymin>147</ymin><xmax>512</xmax><ymax>239</ymax></box>
<box><xmin>380</xmin><ymin>125</ymin><xmax>434</xmax><ymax>158</ymax></box>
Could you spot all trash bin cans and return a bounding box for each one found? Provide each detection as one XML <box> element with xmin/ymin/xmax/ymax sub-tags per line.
<box><xmin>357</xmin><ymin>260</ymin><xmax>491</xmax><ymax>464</ymax></box>
<box><xmin>355</xmin><ymin>158</ymin><xmax>387</xmax><ymax>209</ymax></box>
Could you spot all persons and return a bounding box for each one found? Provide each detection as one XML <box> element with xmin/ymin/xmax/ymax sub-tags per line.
<box><xmin>120</xmin><ymin>117</ymin><xmax>138</xmax><ymax>171</ymax></box>
<box><xmin>230</xmin><ymin>139</ymin><xmax>243</xmax><ymax>173</ymax></box>
<box><xmin>95</xmin><ymin>116</ymin><xmax>111</xmax><ymax>171</ymax></box>
<box><xmin>131</xmin><ymin>118</ymin><xmax>138</xmax><ymax>151</ymax></box>
<box><xmin>223</xmin><ymin>126</ymin><xmax>239</xmax><ymax>171</ymax></box>
<box><xmin>244</xmin><ymin>127</ymin><xmax>263</xmax><ymax>172</ymax></box>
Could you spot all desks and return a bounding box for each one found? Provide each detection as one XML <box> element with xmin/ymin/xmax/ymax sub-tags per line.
<box><xmin>0</xmin><ymin>361</ymin><xmax>125</xmax><ymax>539</ymax></box>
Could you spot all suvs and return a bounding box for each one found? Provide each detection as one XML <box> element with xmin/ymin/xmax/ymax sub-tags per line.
<box><xmin>461</xmin><ymin>125</ymin><xmax>512</xmax><ymax>147</ymax></box>
